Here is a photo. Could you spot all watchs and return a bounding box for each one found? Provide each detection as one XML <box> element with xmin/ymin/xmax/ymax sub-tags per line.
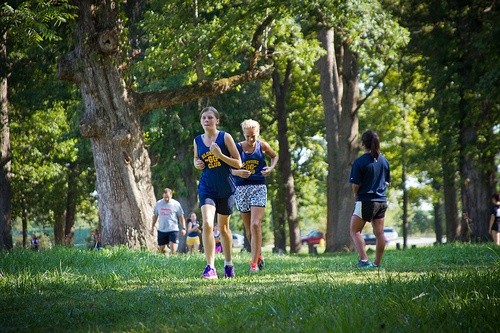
<box><xmin>183</xmin><ymin>228</ymin><xmax>185</xmax><ymax>230</ymax></box>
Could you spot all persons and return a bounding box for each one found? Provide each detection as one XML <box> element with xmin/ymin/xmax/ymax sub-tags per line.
<box><xmin>151</xmin><ymin>188</ymin><xmax>187</xmax><ymax>256</ymax></box>
<box><xmin>231</xmin><ymin>119</ymin><xmax>279</xmax><ymax>271</ymax></box>
<box><xmin>193</xmin><ymin>106</ymin><xmax>243</xmax><ymax>278</ymax></box>
<box><xmin>488</xmin><ymin>193</ymin><xmax>500</xmax><ymax>245</ymax></box>
<box><xmin>187</xmin><ymin>212</ymin><xmax>223</xmax><ymax>254</ymax></box>
<box><xmin>349</xmin><ymin>129</ymin><xmax>392</xmax><ymax>267</ymax></box>
<box><xmin>94</xmin><ymin>230</ymin><xmax>100</xmax><ymax>249</ymax></box>
<box><xmin>31</xmin><ymin>233</ymin><xmax>50</xmax><ymax>251</ymax></box>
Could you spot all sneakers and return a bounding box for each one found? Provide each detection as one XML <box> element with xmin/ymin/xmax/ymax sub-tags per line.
<box><xmin>257</xmin><ymin>255</ymin><xmax>265</xmax><ymax>270</ymax></box>
<box><xmin>354</xmin><ymin>260</ymin><xmax>370</xmax><ymax>268</ymax></box>
<box><xmin>224</xmin><ymin>264</ymin><xmax>235</xmax><ymax>279</ymax></box>
<box><xmin>250</xmin><ymin>261</ymin><xmax>259</xmax><ymax>273</ymax></box>
<box><xmin>370</xmin><ymin>264</ymin><xmax>381</xmax><ymax>267</ymax></box>
<box><xmin>203</xmin><ymin>264</ymin><xmax>218</xmax><ymax>279</ymax></box>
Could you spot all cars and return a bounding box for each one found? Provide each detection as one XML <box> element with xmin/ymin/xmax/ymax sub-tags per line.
<box><xmin>361</xmin><ymin>233</ymin><xmax>389</xmax><ymax>246</ymax></box>
<box><xmin>230</xmin><ymin>234</ymin><xmax>245</xmax><ymax>247</ymax></box>
<box><xmin>300</xmin><ymin>230</ymin><xmax>325</xmax><ymax>245</ymax></box>
<box><xmin>369</xmin><ymin>228</ymin><xmax>399</xmax><ymax>241</ymax></box>
